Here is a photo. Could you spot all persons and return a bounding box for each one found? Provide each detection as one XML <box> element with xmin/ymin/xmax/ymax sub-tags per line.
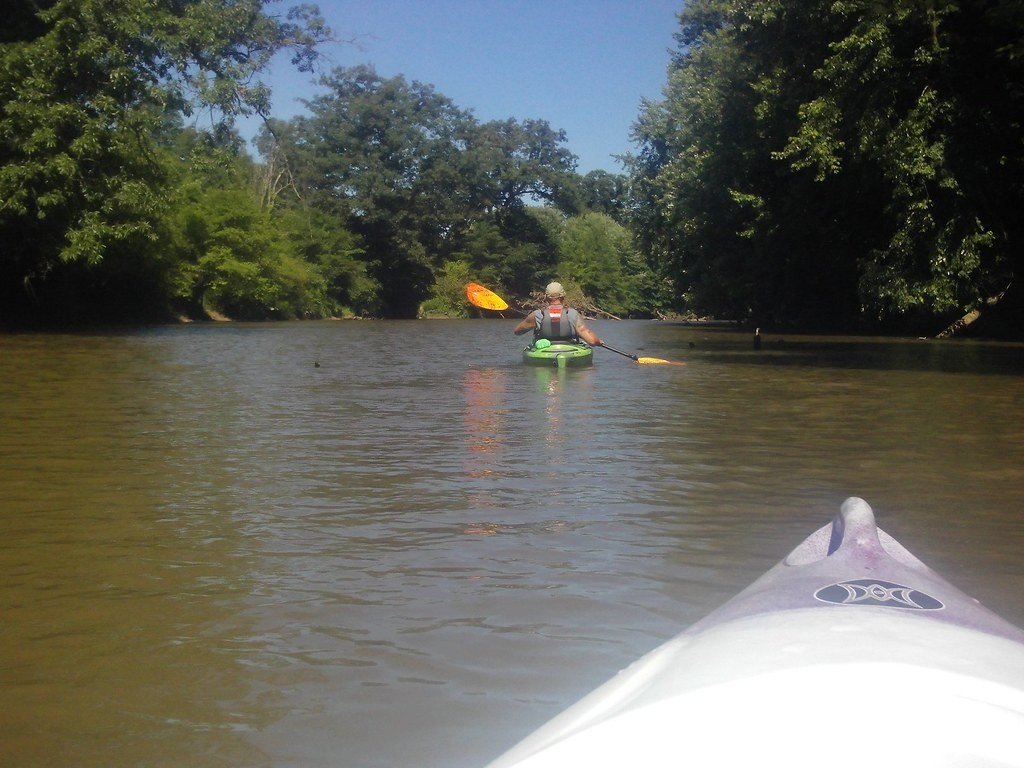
<box><xmin>514</xmin><ymin>282</ymin><xmax>604</xmax><ymax>347</ymax></box>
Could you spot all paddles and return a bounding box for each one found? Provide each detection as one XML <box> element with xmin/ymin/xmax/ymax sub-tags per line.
<box><xmin>464</xmin><ymin>283</ymin><xmax>686</xmax><ymax>366</ymax></box>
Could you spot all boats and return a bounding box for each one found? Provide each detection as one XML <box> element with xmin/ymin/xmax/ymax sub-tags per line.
<box><xmin>523</xmin><ymin>338</ymin><xmax>594</xmax><ymax>367</ymax></box>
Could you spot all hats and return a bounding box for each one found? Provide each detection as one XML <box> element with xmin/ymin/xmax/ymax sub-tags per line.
<box><xmin>545</xmin><ymin>282</ymin><xmax>566</xmax><ymax>299</ymax></box>
